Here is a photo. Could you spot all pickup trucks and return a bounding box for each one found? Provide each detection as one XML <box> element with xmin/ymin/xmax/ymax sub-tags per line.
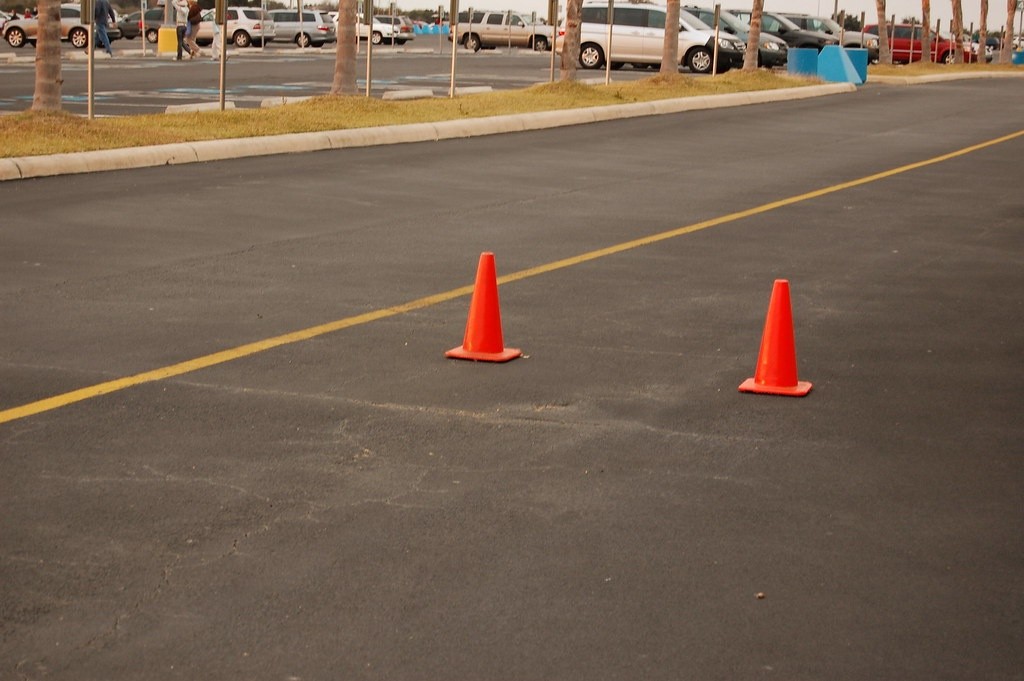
<box><xmin>449</xmin><ymin>10</ymin><xmax>559</xmax><ymax>53</ymax></box>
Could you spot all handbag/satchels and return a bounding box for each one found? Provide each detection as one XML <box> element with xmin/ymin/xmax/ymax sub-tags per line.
<box><xmin>184</xmin><ymin>19</ymin><xmax>193</xmax><ymax>38</ymax></box>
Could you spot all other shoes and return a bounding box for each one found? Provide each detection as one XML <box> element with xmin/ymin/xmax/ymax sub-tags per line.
<box><xmin>190</xmin><ymin>50</ymin><xmax>195</xmax><ymax>59</ymax></box>
<box><xmin>173</xmin><ymin>57</ymin><xmax>182</xmax><ymax>61</ymax></box>
<box><xmin>83</xmin><ymin>50</ymin><xmax>90</xmax><ymax>56</ymax></box>
<box><xmin>225</xmin><ymin>55</ymin><xmax>231</xmax><ymax>61</ymax></box>
<box><xmin>210</xmin><ymin>58</ymin><xmax>219</xmax><ymax>61</ymax></box>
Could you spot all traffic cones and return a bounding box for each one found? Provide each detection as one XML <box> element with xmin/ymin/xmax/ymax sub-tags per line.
<box><xmin>737</xmin><ymin>278</ymin><xmax>813</xmax><ymax>398</ymax></box>
<box><xmin>444</xmin><ymin>252</ymin><xmax>523</xmax><ymax>363</ymax></box>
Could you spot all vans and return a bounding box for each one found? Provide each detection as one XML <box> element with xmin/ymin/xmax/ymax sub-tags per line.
<box><xmin>267</xmin><ymin>9</ymin><xmax>337</xmax><ymax>48</ymax></box>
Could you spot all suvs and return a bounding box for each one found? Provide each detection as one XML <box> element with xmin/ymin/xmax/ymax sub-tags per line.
<box><xmin>862</xmin><ymin>22</ymin><xmax>994</xmax><ymax>65</ymax></box>
<box><xmin>196</xmin><ymin>6</ymin><xmax>276</xmax><ymax>47</ymax></box>
<box><xmin>138</xmin><ymin>7</ymin><xmax>178</xmax><ymax>44</ymax></box>
<box><xmin>555</xmin><ymin>1</ymin><xmax>745</xmax><ymax>74</ymax></box>
<box><xmin>2</xmin><ymin>3</ymin><xmax>121</xmax><ymax>48</ymax></box>
<box><xmin>397</xmin><ymin>16</ymin><xmax>416</xmax><ymax>45</ymax></box>
<box><xmin>762</xmin><ymin>12</ymin><xmax>880</xmax><ymax>65</ymax></box>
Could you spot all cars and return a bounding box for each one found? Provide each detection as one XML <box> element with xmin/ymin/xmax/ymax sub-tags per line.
<box><xmin>327</xmin><ymin>11</ymin><xmax>400</xmax><ymax>45</ymax></box>
<box><xmin>681</xmin><ymin>7</ymin><xmax>791</xmax><ymax>68</ymax></box>
<box><xmin>701</xmin><ymin>8</ymin><xmax>840</xmax><ymax>54</ymax></box>
<box><xmin>976</xmin><ymin>36</ymin><xmax>1024</xmax><ymax>49</ymax></box>
<box><xmin>0</xmin><ymin>10</ymin><xmax>36</xmax><ymax>30</ymax></box>
<box><xmin>412</xmin><ymin>19</ymin><xmax>425</xmax><ymax>28</ymax></box>
<box><xmin>116</xmin><ymin>10</ymin><xmax>141</xmax><ymax>40</ymax></box>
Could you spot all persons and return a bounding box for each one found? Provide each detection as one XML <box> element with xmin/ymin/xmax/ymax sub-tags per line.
<box><xmin>185</xmin><ymin>0</ymin><xmax>205</xmax><ymax>59</ymax></box>
<box><xmin>84</xmin><ymin>0</ymin><xmax>117</xmax><ymax>57</ymax></box>
<box><xmin>32</xmin><ymin>7</ymin><xmax>39</xmax><ymax>16</ymax></box>
<box><xmin>210</xmin><ymin>26</ymin><xmax>230</xmax><ymax>62</ymax></box>
<box><xmin>171</xmin><ymin>0</ymin><xmax>194</xmax><ymax>61</ymax></box>
<box><xmin>10</xmin><ymin>9</ymin><xmax>20</xmax><ymax>20</ymax></box>
<box><xmin>24</xmin><ymin>8</ymin><xmax>31</xmax><ymax>18</ymax></box>
